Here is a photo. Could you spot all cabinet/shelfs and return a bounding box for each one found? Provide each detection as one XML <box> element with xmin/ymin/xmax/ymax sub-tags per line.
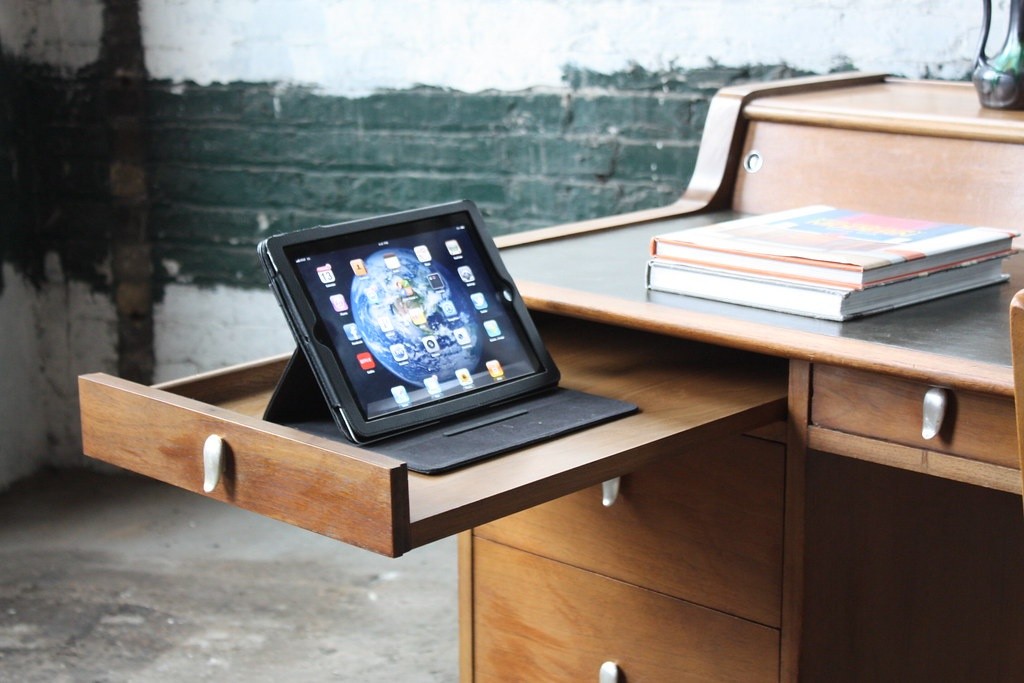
<box><xmin>78</xmin><ymin>73</ymin><xmax>1024</xmax><ymax>683</ymax></box>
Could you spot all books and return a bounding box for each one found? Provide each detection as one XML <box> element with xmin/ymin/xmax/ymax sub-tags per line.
<box><xmin>645</xmin><ymin>202</ymin><xmax>1023</xmax><ymax>322</ymax></box>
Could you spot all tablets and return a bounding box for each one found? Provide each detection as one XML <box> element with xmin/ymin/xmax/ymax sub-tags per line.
<box><xmin>263</xmin><ymin>199</ymin><xmax>561</xmax><ymax>449</ymax></box>
